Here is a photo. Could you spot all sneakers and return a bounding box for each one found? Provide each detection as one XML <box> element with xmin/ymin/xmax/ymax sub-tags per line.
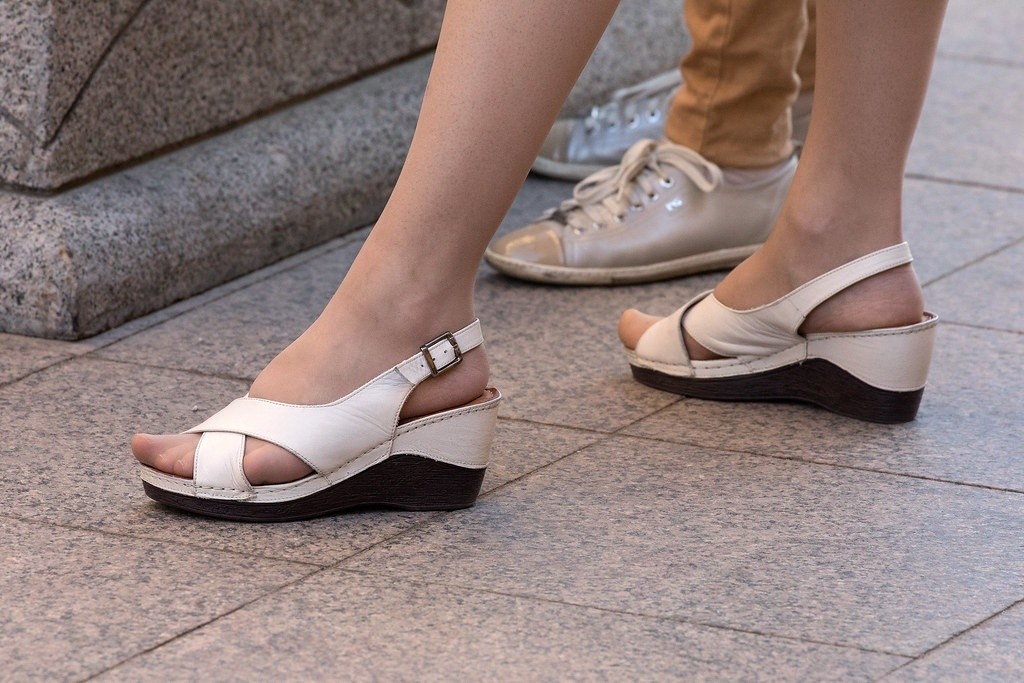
<box><xmin>482</xmin><ymin>137</ymin><xmax>800</xmax><ymax>284</ymax></box>
<box><xmin>529</xmin><ymin>68</ymin><xmax>813</xmax><ymax>179</ymax></box>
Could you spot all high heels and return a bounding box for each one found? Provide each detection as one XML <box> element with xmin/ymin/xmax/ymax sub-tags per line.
<box><xmin>622</xmin><ymin>241</ymin><xmax>939</xmax><ymax>425</ymax></box>
<box><xmin>138</xmin><ymin>318</ymin><xmax>502</xmax><ymax>523</ymax></box>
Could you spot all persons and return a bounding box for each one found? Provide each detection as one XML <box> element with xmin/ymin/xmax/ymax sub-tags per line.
<box><xmin>484</xmin><ymin>0</ymin><xmax>814</xmax><ymax>282</ymax></box>
<box><xmin>134</xmin><ymin>0</ymin><xmax>945</xmax><ymax>524</ymax></box>
